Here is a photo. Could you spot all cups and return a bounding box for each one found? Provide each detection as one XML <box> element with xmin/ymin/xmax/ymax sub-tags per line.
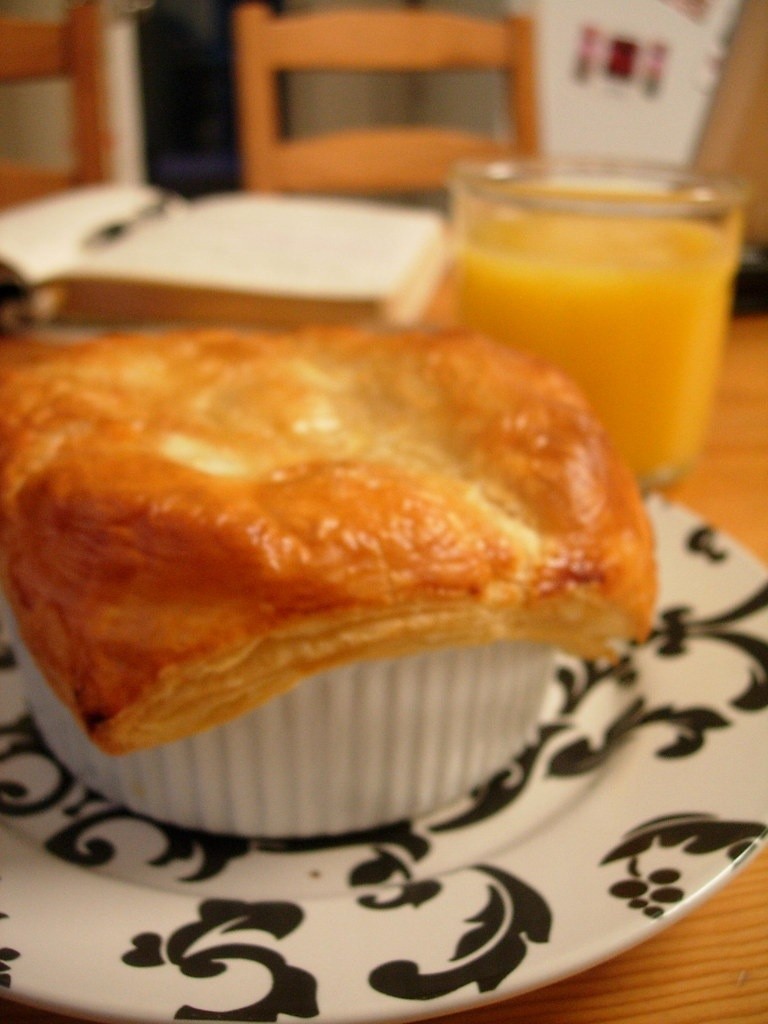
<box><xmin>447</xmin><ymin>153</ymin><xmax>752</xmax><ymax>496</ymax></box>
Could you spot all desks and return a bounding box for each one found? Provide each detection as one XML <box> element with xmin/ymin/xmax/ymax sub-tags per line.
<box><xmin>0</xmin><ymin>314</ymin><xmax>768</xmax><ymax>1024</ymax></box>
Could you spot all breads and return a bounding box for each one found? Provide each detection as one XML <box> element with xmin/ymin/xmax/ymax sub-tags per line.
<box><xmin>0</xmin><ymin>315</ymin><xmax>658</xmax><ymax>750</ymax></box>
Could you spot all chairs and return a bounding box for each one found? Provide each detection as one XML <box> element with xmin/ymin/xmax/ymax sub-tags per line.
<box><xmin>234</xmin><ymin>2</ymin><xmax>537</xmax><ymax>196</ymax></box>
<box><xmin>0</xmin><ymin>0</ymin><xmax>113</xmax><ymax>210</ymax></box>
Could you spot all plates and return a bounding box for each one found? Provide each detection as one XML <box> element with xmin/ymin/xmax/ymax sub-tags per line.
<box><xmin>0</xmin><ymin>494</ymin><xmax>768</xmax><ymax>1024</ymax></box>
